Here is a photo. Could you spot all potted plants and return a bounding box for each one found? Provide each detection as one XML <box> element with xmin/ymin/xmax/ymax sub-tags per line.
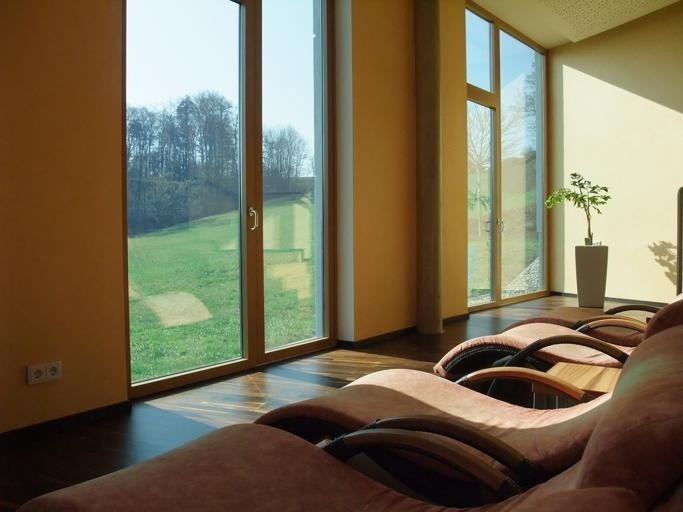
<box><xmin>543</xmin><ymin>172</ymin><xmax>612</xmax><ymax>308</ymax></box>
